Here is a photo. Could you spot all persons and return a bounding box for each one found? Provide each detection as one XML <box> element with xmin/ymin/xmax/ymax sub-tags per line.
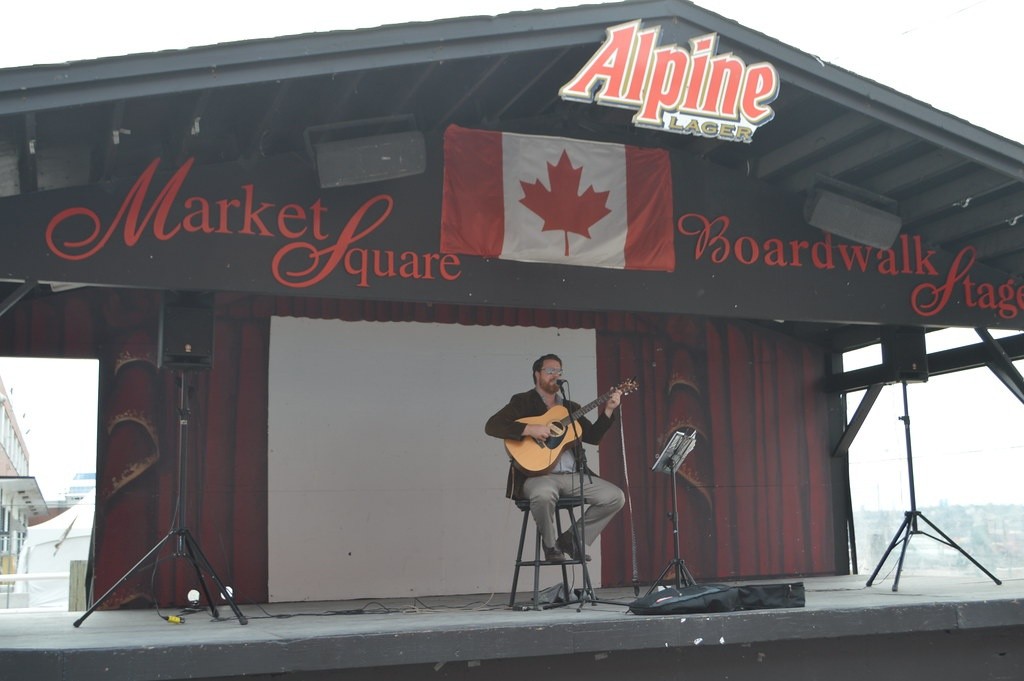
<box><xmin>485</xmin><ymin>354</ymin><xmax>625</xmax><ymax>563</ymax></box>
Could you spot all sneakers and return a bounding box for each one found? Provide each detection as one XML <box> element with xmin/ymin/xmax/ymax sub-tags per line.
<box><xmin>555</xmin><ymin>530</ymin><xmax>591</xmax><ymax>562</ymax></box>
<box><xmin>541</xmin><ymin>540</ymin><xmax>565</xmax><ymax>563</ymax></box>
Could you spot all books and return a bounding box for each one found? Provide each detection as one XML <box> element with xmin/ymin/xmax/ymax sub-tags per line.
<box><xmin>652</xmin><ymin>431</ymin><xmax>697</xmax><ymax>474</ymax></box>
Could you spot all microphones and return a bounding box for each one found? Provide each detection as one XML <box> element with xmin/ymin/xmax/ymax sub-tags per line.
<box><xmin>556</xmin><ymin>378</ymin><xmax>567</xmax><ymax>386</ymax></box>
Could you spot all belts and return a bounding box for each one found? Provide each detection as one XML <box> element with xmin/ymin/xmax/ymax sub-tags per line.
<box><xmin>553</xmin><ymin>470</ymin><xmax>573</xmax><ymax>475</ymax></box>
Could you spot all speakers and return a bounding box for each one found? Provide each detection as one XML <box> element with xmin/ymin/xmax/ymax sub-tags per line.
<box><xmin>801</xmin><ymin>188</ymin><xmax>901</xmax><ymax>250</ymax></box>
<box><xmin>315</xmin><ymin>130</ymin><xmax>427</xmax><ymax>188</ymax></box>
<box><xmin>880</xmin><ymin>325</ymin><xmax>928</xmax><ymax>385</ymax></box>
<box><xmin>163</xmin><ymin>289</ymin><xmax>215</xmax><ymax>370</ymax></box>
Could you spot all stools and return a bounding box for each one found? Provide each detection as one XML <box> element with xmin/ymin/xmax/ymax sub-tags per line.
<box><xmin>508</xmin><ymin>497</ymin><xmax>598</xmax><ymax>609</ymax></box>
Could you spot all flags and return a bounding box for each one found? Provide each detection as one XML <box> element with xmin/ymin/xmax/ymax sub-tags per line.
<box><xmin>440</xmin><ymin>123</ymin><xmax>675</xmax><ymax>273</ymax></box>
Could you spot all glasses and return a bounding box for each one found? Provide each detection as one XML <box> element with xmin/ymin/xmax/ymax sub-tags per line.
<box><xmin>539</xmin><ymin>367</ymin><xmax>565</xmax><ymax>377</ymax></box>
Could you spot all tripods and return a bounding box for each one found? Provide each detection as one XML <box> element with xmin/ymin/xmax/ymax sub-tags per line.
<box><xmin>542</xmin><ymin>386</ymin><xmax>630</xmax><ymax>615</ymax></box>
<box><xmin>73</xmin><ymin>369</ymin><xmax>248</xmax><ymax>627</ymax></box>
<box><xmin>865</xmin><ymin>380</ymin><xmax>1002</xmax><ymax>592</ymax></box>
<box><xmin>641</xmin><ymin>433</ymin><xmax>698</xmax><ymax>600</ymax></box>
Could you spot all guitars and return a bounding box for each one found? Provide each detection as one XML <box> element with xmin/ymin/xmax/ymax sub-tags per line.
<box><xmin>505</xmin><ymin>377</ymin><xmax>640</xmax><ymax>475</ymax></box>
